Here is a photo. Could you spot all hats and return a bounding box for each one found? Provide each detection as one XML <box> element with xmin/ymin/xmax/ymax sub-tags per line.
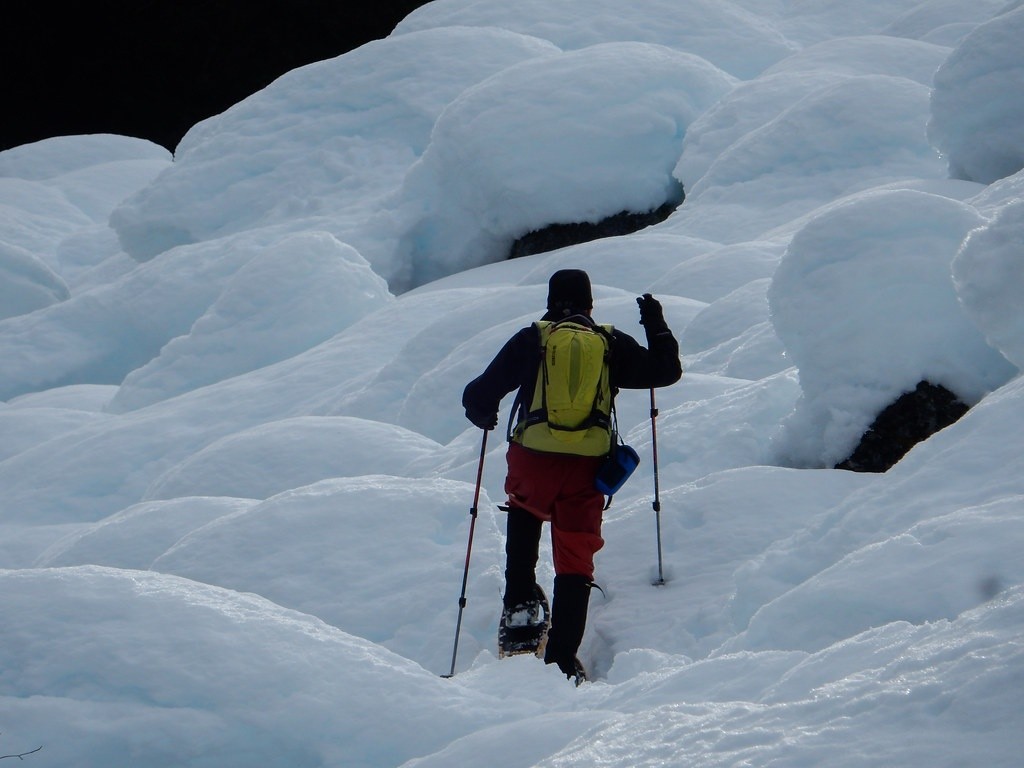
<box><xmin>547</xmin><ymin>269</ymin><xmax>593</xmax><ymax>309</ymax></box>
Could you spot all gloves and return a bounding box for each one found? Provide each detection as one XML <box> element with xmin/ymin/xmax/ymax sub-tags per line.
<box><xmin>636</xmin><ymin>294</ymin><xmax>663</xmax><ymax>324</ymax></box>
<box><xmin>462</xmin><ymin>375</ymin><xmax>500</xmax><ymax>430</ymax></box>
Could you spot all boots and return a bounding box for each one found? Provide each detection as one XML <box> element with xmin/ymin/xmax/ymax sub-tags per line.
<box><xmin>544</xmin><ymin>574</ymin><xmax>605</xmax><ymax>688</ymax></box>
<box><xmin>499</xmin><ymin>569</ymin><xmax>551</xmax><ymax>660</ymax></box>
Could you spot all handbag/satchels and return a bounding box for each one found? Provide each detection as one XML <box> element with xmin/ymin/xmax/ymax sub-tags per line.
<box><xmin>594</xmin><ymin>445</ymin><xmax>641</xmax><ymax>496</ymax></box>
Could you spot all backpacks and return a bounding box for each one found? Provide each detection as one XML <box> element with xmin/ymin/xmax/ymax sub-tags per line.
<box><xmin>506</xmin><ymin>315</ymin><xmax>619</xmax><ymax>456</ymax></box>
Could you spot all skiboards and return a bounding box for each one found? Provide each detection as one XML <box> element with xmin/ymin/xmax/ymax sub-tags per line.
<box><xmin>496</xmin><ymin>582</ymin><xmax>588</xmax><ymax>689</ymax></box>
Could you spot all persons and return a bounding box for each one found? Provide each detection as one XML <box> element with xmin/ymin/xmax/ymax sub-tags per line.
<box><xmin>462</xmin><ymin>269</ymin><xmax>682</xmax><ymax>685</ymax></box>
